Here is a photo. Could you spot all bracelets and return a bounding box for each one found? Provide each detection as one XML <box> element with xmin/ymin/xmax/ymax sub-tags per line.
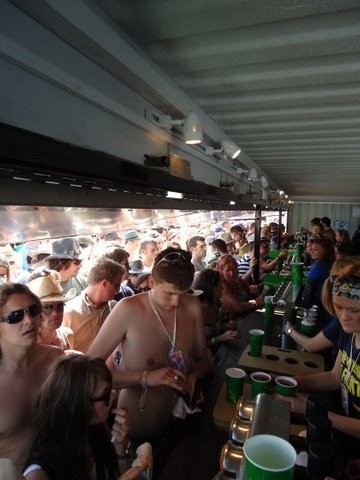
<box><xmin>142</xmin><ymin>370</ymin><xmax>152</xmax><ymax>389</ymax></box>
<box><xmin>211</xmin><ymin>337</ymin><xmax>216</xmax><ymax>344</ymax></box>
<box><xmin>250</xmin><ymin>300</ymin><xmax>257</xmax><ymax>307</ymax></box>
<box><xmin>296</xmin><ymin>376</ymin><xmax>302</xmax><ymax>389</ymax></box>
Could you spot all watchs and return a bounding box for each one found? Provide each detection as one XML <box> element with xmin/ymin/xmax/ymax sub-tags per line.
<box><xmin>288</xmin><ymin>328</ymin><xmax>294</xmax><ymax>334</ymax></box>
<box><xmin>116</xmin><ymin>440</ymin><xmax>133</xmax><ymax>459</ymax></box>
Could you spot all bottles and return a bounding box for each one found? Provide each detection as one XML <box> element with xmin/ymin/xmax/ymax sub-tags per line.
<box><xmin>294</xmin><ymin>238</ymin><xmax>302</xmax><ymax>262</ymax></box>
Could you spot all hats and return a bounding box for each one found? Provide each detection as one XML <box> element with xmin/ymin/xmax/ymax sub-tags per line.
<box><xmin>105</xmin><ymin>232</ymin><xmax>121</xmax><ymax>241</ymax></box>
<box><xmin>239</xmin><ymin>231</ymin><xmax>269</xmax><ymax>255</ymax></box>
<box><xmin>26</xmin><ymin>275</ymin><xmax>77</xmax><ymax>303</ymax></box>
<box><xmin>45</xmin><ymin>237</ymin><xmax>89</xmax><ymax>261</ymax></box>
<box><xmin>166</xmin><ymin>227</ymin><xmax>180</xmax><ymax>240</ymax></box>
<box><xmin>123</xmin><ymin>231</ymin><xmax>139</xmax><ymax>242</ymax></box>
<box><xmin>220</xmin><ymin>233</ymin><xmax>236</xmax><ymax>245</ymax></box>
<box><xmin>29</xmin><ymin>243</ymin><xmax>52</xmax><ymax>260</ymax></box>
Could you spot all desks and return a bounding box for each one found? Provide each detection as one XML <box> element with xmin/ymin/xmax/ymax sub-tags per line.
<box><xmin>154</xmin><ymin>303</ymin><xmax>331</xmax><ymax>480</ymax></box>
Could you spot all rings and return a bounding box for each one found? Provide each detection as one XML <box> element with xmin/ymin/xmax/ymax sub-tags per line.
<box><xmin>175</xmin><ymin>375</ymin><xmax>179</xmax><ymax>380</ymax></box>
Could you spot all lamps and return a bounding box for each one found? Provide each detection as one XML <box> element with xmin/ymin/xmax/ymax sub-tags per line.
<box><xmin>203</xmin><ymin>138</ymin><xmax>242</xmax><ymax>159</ymax></box>
<box><xmin>253</xmin><ymin>176</ymin><xmax>269</xmax><ymax>188</ymax></box>
<box><xmin>236</xmin><ymin>167</ymin><xmax>257</xmax><ymax>181</ymax></box>
<box><xmin>270</xmin><ymin>188</ymin><xmax>285</xmax><ymax>196</ymax></box>
<box><xmin>159</xmin><ymin>111</ymin><xmax>204</xmax><ymax>145</ymax></box>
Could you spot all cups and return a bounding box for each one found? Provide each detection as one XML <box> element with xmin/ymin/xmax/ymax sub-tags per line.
<box><xmin>263</xmin><ymin>295</ymin><xmax>276</xmax><ymax>322</ymax></box>
<box><xmin>249</xmin><ymin>329</ymin><xmax>265</xmax><ymax>357</ymax></box>
<box><xmin>274</xmin><ymin>375</ymin><xmax>298</xmax><ymax>398</ymax></box>
<box><xmin>250</xmin><ymin>371</ymin><xmax>271</xmax><ymax>401</ymax></box>
<box><xmin>282</xmin><ymin>307</ymin><xmax>296</xmax><ymax>350</ymax></box>
<box><xmin>293</xmin><ymin>262</ymin><xmax>304</xmax><ymax>285</ymax></box>
<box><xmin>226</xmin><ymin>367</ymin><xmax>246</xmax><ymax>405</ymax></box>
<box><xmin>242</xmin><ymin>433</ymin><xmax>297</xmax><ymax>480</ymax></box>
<box><xmin>301</xmin><ymin>320</ymin><xmax>316</xmax><ymax>338</ymax></box>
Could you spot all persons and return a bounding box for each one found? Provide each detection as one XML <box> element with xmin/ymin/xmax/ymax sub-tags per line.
<box><xmin>87</xmin><ymin>253</ymin><xmax>214</xmax><ymax>480</ymax></box>
<box><xmin>260</xmin><ymin>268</ymin><xmax>360</xmax><ymax>480</ymax></box>
<box><xmin>0</xmin><ymin>217</ymin><xmax>360</xmax><ymax>424</ymax></box>
<box><xmin>0</xmin><ymin>283</ymin><xmax>65</xmax><ymax>469</ymax></box>
<box><xmin>22</xmin><ymin>353</ymin><xmax>149</xmax><ymax>480</ymax></box>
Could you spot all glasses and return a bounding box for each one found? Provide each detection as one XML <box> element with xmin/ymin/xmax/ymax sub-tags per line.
<box><xmin>328</xmin><ymin>273</ymin><xmax>360</xmax><ymax>289</ymax></box>
<box><xmin>40</xmin><ymin>302</ymin><xmax>66</xmax><ymax>315</ymax></box>
<box><xmin>0</xmin><ymin>304</ymin><xmax>41</xmax><ymax>325</ymax></box>
<box><xmin>156</xmin><ymin>251</ymin><xmax>193</xmax><ymax>267</ymax></box>
<box><xmin>262</xmin><ymin>243</ymin><xmax>270</xmax><ymax>248</ymax></box>
<box><xmin>86</xmin><ymin>385</ymin><xmax>113</xmax><ymax>407</ymax></box>
<box><xmin>70</xmin><ymin>259</ymin><xmax>83</xmax><ymax>265</ymax></box>
